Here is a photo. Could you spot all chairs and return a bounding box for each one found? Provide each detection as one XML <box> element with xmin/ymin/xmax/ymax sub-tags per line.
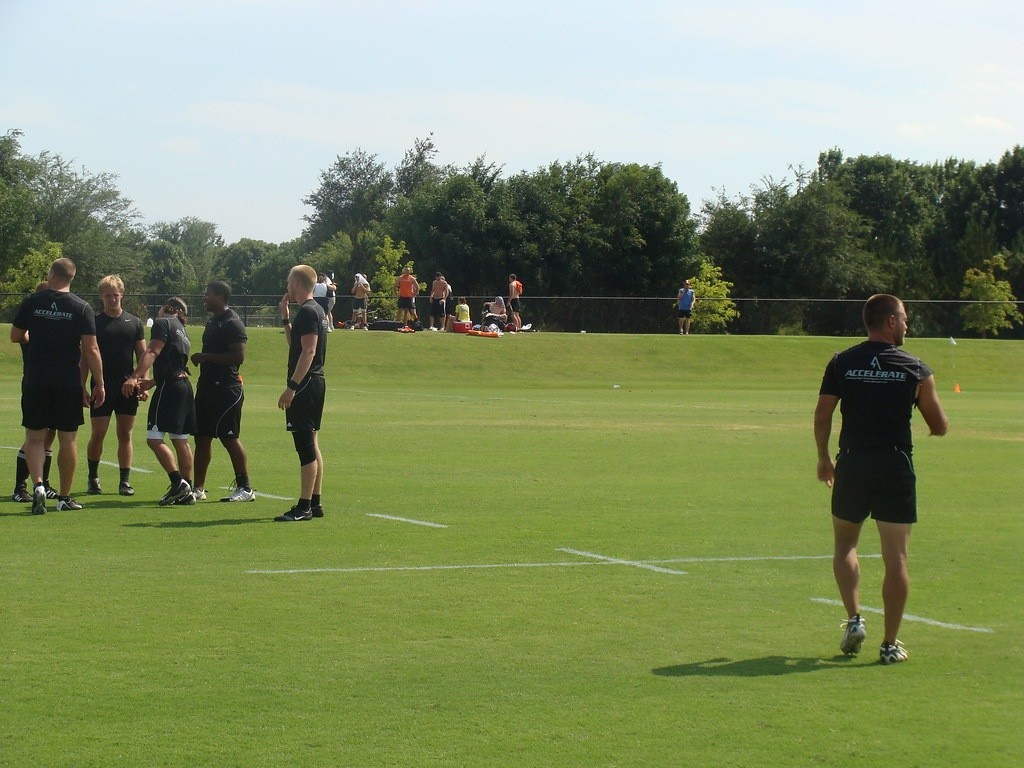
<box><xmin>482</xmin><ymin>305</ymin><xmax>506</xmax><ymax>331</ymax></box>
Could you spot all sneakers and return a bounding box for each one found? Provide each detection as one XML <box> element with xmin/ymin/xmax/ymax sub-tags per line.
<box><xmin>46</xmin><ymin>487</ymin><xmax>61</xmax><ymax>499</ymax></box>
<box><xmin>397</xmin><ymin>326</ymin><xmax>414</xmax><ymax>333</ymax></box>
<box><xmin>87</xmin><ymin>474</ymin><xmax>102</xmax><ymax>495</ymax></box>
<box><xmin>159</xmin><ymin>478</ymin><xmax>196</xmax><ymax>506</ymax></box>
<box><xmin>312</xmin><ymin>504</ymin><xmax>323</xmax><ymax>517</ymax></box>
<box><xmin>220</xmin><ymin>486</ymin><xmax>255</xmax><ymax>502</ymax></box>
<box><xmin>119</xmin><ymin>482</ymin><xmax>134</xmax><ymax>495</ymax></box>
<box><xmin>839</xmin><ymin>613</ymin><xmax>867</xmax><ymax>655</ymax></box>
<box><xmin>12</xmin><ymin>490</ymin><xmax>34</xmax><ymax>502</ymax></box>
<box><xmin>56</xmin><ymin>496</ymin><xmax>83</xmax><ymax>511</ymax></box>
<box><xmin>31</xmin><ymin>485</ymin><xmax>47</xmax><ymax>515</ymax></box>
<box><xmin>880</xmin><ymin>640</ymin><xmax>908</xmax><ymax>665</ymax></box>
<box><xmin>192</xmin><ymin>489</ymin><xmax>207</xmax><ymax>499</ymax></box>
<box><xmin>274</xmin><ymin>504</ymin><xmax>313</xmax><ymax>521</ymax></box>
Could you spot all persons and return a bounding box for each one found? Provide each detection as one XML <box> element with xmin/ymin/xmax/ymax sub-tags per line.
<box><xmin>678</xmin><ymin>280</ymin><xmax>695</xmax><ymax>335</ymax></box>
<box><xmin>350</xmin><ymin>273</ymin><xmax>370</xmax><ymax>331</ymax></box>
<box><xmin>122</xmin><ymin>297</ymin><xmax>196</xmax><ymax>505</ymax></box>
<box><xmin>10</xmin><ymin>259</ymin><xmax>106</xmax><ymax>515</ymax></box>
<box><xmin>190</xmin><ymin>281</ymin><xmax>255</xmax><ymax>502</ymax></box>
<box><xmin>394</xmin><ymin>266</ymin><xmax>420</xmax><ymax>328</ymax></box>
<box><xmin>814</xmin><ymin>294</ymin><xmax>947</xmax><ymax>662</ymax></box>
<box><xmin>274</xmin><ymin>264</ymin><xmax>327</xmax><ymax>522</ymax></box>
<box><xmin>80</xmin><ymin>275</ymin><xmax>148</xmax><ymax>496</ymax></box>
<box><xmin>507</xmin><ymin>274</ymin><xmax>522</xmax><ymax>332</ymax></box>
<box><xmin>429</xmin><ymin>272</ymin><xmax>452</xmax><ymax>332</ymax></box>
<box><xmin>449</xmin><ymin>297</ymin><xmax>470</xmax><ymax>333</ymax></box>
<box><xmin>312</xmin><ymin>270</ymin><xmax>337</xmax><ymax>333</ymax></box>
<box><xmin>482</xmin><ymin>296</ymin><xmax>507</xmax><ymax>329</ymax></box>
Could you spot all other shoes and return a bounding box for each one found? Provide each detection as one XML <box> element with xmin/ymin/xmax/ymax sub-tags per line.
<box><xmin>362</xmin><ymin>325</ymin><xmax>368</xmax><ymax>331</ymax></box>
<box><xmin>680</xmin><ymin>329</ymin><xmax>688</xmax><ymax>335</ymax></box>
<box><xmin>327</xmin><ymin>326</ymin><xmax>334</xmax><ymax>333</ymax></box>
<box><xmin>350</xmin><ymin>325</ymin><xmax>354</xmax><ymax>330</ymax></box>
<box><xmin>429</xmin><ymin>325</ymin><xmax>446</xmax><ymax>332</ymax></box>
<box><xmin>414</xmin><ymin>314</ymin><xmax>418</xmax><ymax>322</ymax></box>
<box><xmin>364</xmin><ymin>322</ymin><xmax>369</xmax><ymax>326</ymax></box>
<box><xmin>408</xmin><ymin>318</ymin><xmax>412</xmax><ymax>323</ymax></box>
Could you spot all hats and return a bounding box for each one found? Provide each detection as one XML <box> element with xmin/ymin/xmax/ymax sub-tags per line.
<box><xmin>496</xmin><ymin>296</ymin><xmax>505</xmax><ymax>308</ymax></box>
<box><xmin>434</xmin><ymin>272</ymin><xmax>442</xmax><ymax>276</ymax></box>
<box><xmin>683</xmin><ymin>280</ymin><xmax>691</xmax><ymax>285</ymax></box>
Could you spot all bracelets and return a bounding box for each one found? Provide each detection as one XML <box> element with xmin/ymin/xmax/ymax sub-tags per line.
<box><xmin>288</xmin><ymin>380</ymin><xmax>300</xmax><ymax>390</ymax></box>
<box><xmin>281</xmin><ymin>318</ymin><xmax>291</xmax><ymax>325</ymax></box>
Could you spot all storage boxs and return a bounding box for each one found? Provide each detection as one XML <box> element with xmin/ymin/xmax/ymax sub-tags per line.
<box><xmin>453</xmin><ymin>321</ymin><xmax>472</xmax><ymax>333</ymax></box>
